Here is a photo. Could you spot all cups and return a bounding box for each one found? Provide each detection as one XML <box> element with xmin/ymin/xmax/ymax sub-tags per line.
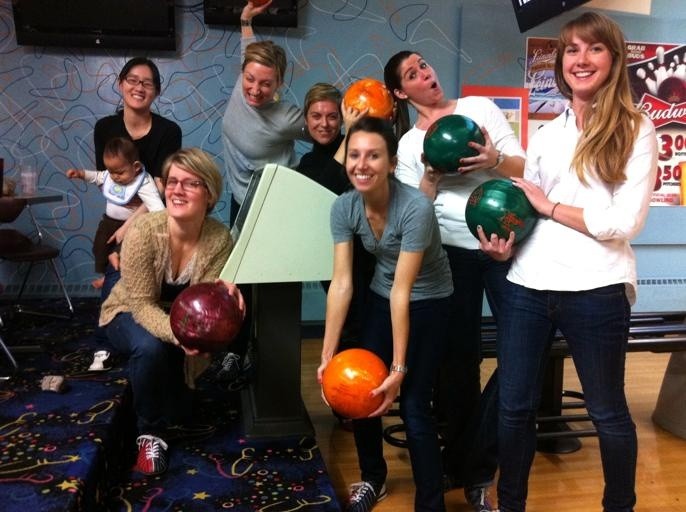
<box><xmin>120</xmin><ymin>75</ymin><xmax>158</xmax><ymax>90</ymax></box>
<box><xmin>163</xmin><ymin>178</ymin><xmax>210</xmax><ymax>191</ymax></box>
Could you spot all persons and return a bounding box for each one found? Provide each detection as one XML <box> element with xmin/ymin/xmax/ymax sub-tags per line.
<box><xmin>222</xmin><ymin>1</ymin><xmax>314</xmax><ymax>231</ymax></box>
<box><xmin>99</xmin><ymin>147</ymin><xmax>246</xmax><ymax>477</ymax></box>
<box><xmin>317</xmin><ymin>117</ymin><xmax>456</xmax><ymax>508</ymax></box>
<box><xmin>293</xmin><ymin>83</ymin><xmax>400</xmax><ymax>432</ymax></box>
<box><xmin>63</xmin><ymin>58</ymin><xmax>183</xmax><ymax>373</ymax></box>
<box><xmin>476</xmin><ymin>13</ymin><xmax>658</xmax><ymax>509</ymax></box>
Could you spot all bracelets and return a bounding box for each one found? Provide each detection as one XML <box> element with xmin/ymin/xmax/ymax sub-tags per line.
<box><xmin>390</xmin><ymin>364</ymin><xmax>408</xmax><ymax>373</ymax></box>
<box><xmin>552</xmin><ymin>202</ymin><xmax>561</xmax><ymax>222</ymax></box>
<box><xmin>241</xmin><ymin>19</ymin><xmax>251</xmax><ymax>27</ymax></box>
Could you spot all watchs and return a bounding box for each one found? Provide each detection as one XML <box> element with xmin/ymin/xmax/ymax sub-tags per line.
<box><xmin>492</xmin><ymin>151</ymin><xmax>504</xmax><ymax>169</ymax></box>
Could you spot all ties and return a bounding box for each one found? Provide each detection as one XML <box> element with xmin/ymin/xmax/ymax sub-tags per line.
<box><xmin>12</xmin><ymin>0</ymin><xmax>179</xmax><ymax>52</ymax></box>
<box><xmin>511</xmin><ymin>0</ymin><xmax>592</xmax><ymax>33</ymax></box>
<box><xmin>203</xmin><ymin>0</ymin><xmax>298</xmax><ymax>28</ymax></box>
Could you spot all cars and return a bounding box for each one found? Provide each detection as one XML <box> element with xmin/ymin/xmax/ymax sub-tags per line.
<box><xmin>18</xmin><ymin>163</ymin><xmax>37</xmax><ymax>195</ymax></box>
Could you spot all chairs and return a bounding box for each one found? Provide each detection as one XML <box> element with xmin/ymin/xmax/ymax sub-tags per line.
<box><xmin>216</xmin><ymin>353</ymin><xmax>242</xmax><ymax>384</ymax></box>
<box><xmin>334</xmin><ymin>414</ymin><xmax>353</xmax><ymax>432</ymax></box>
<box><xmin>135</xmin><ymin>434</ymin><xmax>169</xmax><ymax>476</ymax></box>
<box><xmin>464</xmin><ymin>487</ymin><xmax>492</xmax><ymax>512</ymax></box>
<box><xmin>341</xmin><ymin>482</ymin><xmax>389</xmax><ymax>512</ymax></box>
<box><xmin>90</xmin><ymin>351</ymin><xmax>116</xmax><ymax>372</ymax></box>
<box><xmin>441</xmin><ymin>465</ymin><xmax>496</xmax><ymax>495</ymax></box>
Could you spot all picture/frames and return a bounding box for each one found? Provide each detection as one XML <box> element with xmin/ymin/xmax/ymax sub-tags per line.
<box><xmin>0</xmin><ymin>187</ymin><xmax>63</xmax><ymax>368</ymax></box>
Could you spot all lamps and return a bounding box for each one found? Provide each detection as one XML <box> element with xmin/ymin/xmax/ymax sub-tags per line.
<box><xmin>0</xmin><ymin>157</ymin><xmax>78</xmax><ymax>321</ymax></box>
